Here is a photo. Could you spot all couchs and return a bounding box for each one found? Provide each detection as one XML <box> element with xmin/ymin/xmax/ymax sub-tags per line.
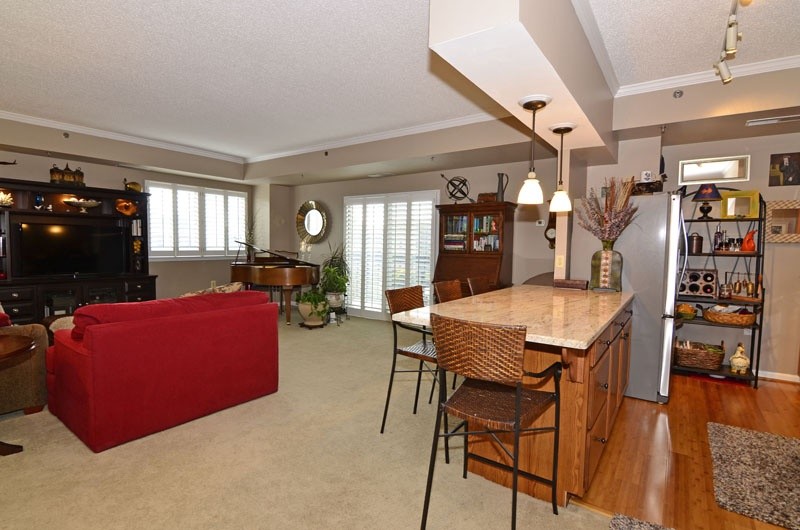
<box><xmin>0</xmin><ymin>305</ymin><xmax>51</xmax><ymax>417</ymax></box>
<box><xmin>46</xmin><ymin>290</ymin><xmax>279</xmax><ymax>456</ymax></box>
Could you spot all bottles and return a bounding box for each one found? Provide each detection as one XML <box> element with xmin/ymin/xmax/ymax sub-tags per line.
<box><xmin>714</xmin><ymin>229</ymin><xmax>744</xmax><ymax>251</ymax></box>
<box><xmin>686</xmin><ymin>232</ymin><xmax>703</xmax><ymax>255</ymax></box>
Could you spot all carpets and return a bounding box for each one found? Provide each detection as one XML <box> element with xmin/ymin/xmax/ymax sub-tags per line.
<box><xmin>606</xmin><ymin>511</ymin><xmax>679</xmax><ymax>530</ymax></box>
<box><xmin>705</xmin><ymin>421</ymin><xmax>800</xmax><ymax>530</ymax></box>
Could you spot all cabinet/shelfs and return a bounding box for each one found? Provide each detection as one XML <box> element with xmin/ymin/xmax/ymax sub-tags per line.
<box><xmin>670</xmin><ymin>190</ymin><xmax>768</xmax><ymax>389</ymax></box>
<box><xmin>429</xmin><ymin>201</ymin><xmax>519</xmax><ymax>305</ymax></box>
<box><xmin>0</xmin><ymin>176</ymin><xmax>160</xmax><ymax>333</ymax></box>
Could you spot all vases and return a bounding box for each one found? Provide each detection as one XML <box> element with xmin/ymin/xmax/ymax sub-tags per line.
<box><xmin>588</xmin><ymin>239</ymin><xmax>625</xmax><ymax>292</ymax></box>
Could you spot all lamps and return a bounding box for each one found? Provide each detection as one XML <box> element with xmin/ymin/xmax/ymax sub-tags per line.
<box><xmin>714</xmin><ymin>52</ymin><xmax>733</xmax><ymax>85</ymax></box>
<box><xmin>721</xmin><ymin>3</ymin><xmax>744</xmax><ymax>54</ymax></box>
<box><xmin>690</xmin><ymin>184</ymin><xmax>723</xmax><ymax>219</ymax></box>
<box><xmin>545</xmin><ymin>122</ymin><xmax>579</xmax><ymax>214</ymax></box>
<box><xmin>515</xmin><ymin>94</ymin><xmax>553</xmax><ymax>205</ymax></box>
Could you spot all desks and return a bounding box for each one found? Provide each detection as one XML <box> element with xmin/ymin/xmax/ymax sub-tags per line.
<box><xmin>425</xmin><ymin>281</ymin><xmax>635</xmax><ymax>507</ymax></box>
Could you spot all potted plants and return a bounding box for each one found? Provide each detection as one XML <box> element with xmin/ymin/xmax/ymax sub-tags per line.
<box><xmin>316</xmin><ymin>242</ymin><xmax>349</xmax><ymax>310</ymax></box>
<box><xmin>293</xmin><ymin>288</ymin><xmax>332</xmax><ymax>329</ymax></box>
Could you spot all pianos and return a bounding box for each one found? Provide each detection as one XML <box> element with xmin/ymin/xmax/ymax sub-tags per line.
<box><xmin>228</xmin><ymin>240</ymin><xmax>320</xmax><ymax>326</ymax></box>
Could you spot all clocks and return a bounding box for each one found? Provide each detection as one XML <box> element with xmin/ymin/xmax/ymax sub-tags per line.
<box><xmin>544</xmin><ymin>199</ymin><xmax>559</xmax><ymax>250</ymax></box>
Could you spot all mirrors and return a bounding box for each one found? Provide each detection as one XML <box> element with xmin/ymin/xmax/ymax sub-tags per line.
<box><xmin>675</xmin><ymin>153</ymin><xmax>753</xmax><ymax>184</ymax></box>
<box><xmin>295</xmin><ymin>200</ymin><xmax>330</xmax><ymax>242</ymax></box>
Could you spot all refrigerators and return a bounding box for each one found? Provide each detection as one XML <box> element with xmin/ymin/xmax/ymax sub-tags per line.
<box><xmin>568</xmin><ymin>193</ymin><xmax>681</xmax><ymax>404</ymax></box>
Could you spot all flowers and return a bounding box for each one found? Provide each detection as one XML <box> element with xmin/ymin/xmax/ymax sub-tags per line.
<box><xmin>572</xmin><ymin>174</ymin><xmax>640</xmax><ymax>242</ymax></box>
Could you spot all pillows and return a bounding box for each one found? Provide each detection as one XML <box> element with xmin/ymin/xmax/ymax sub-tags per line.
<box><xmin>176</xmin><ymin>279</ymin><xmax>244</xmax><ymax>298</ymax></box>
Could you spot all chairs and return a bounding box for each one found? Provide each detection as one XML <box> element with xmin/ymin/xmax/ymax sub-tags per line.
<box><xmin>417</xmin><ymin>311</ymin><xmax>567</xmax><ymax>530</ymax></box>
<box><xmin>467</xmin><ymin>274</ymin><xmax>491</xmax><ymax>299</ymax></box>
<box><xmin>433</xmin><ymin>270</ymin><xmax>464</xmax><ymax>392</ymax></box>
<box><xmin>379</xmin><ymin>286</ymin><xmax>449</xmax><ymax>431</ymax></box>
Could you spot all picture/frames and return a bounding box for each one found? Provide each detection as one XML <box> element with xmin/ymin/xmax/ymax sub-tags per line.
<box><xmin>721</xmin><ymin>189</ymin><xmax>762</xmax><ymax>221</ymax></box>
<box><xmin>761</xmin><ymin>198</ymin><xmax>800</xmax><ymax>244</ymax></box>
